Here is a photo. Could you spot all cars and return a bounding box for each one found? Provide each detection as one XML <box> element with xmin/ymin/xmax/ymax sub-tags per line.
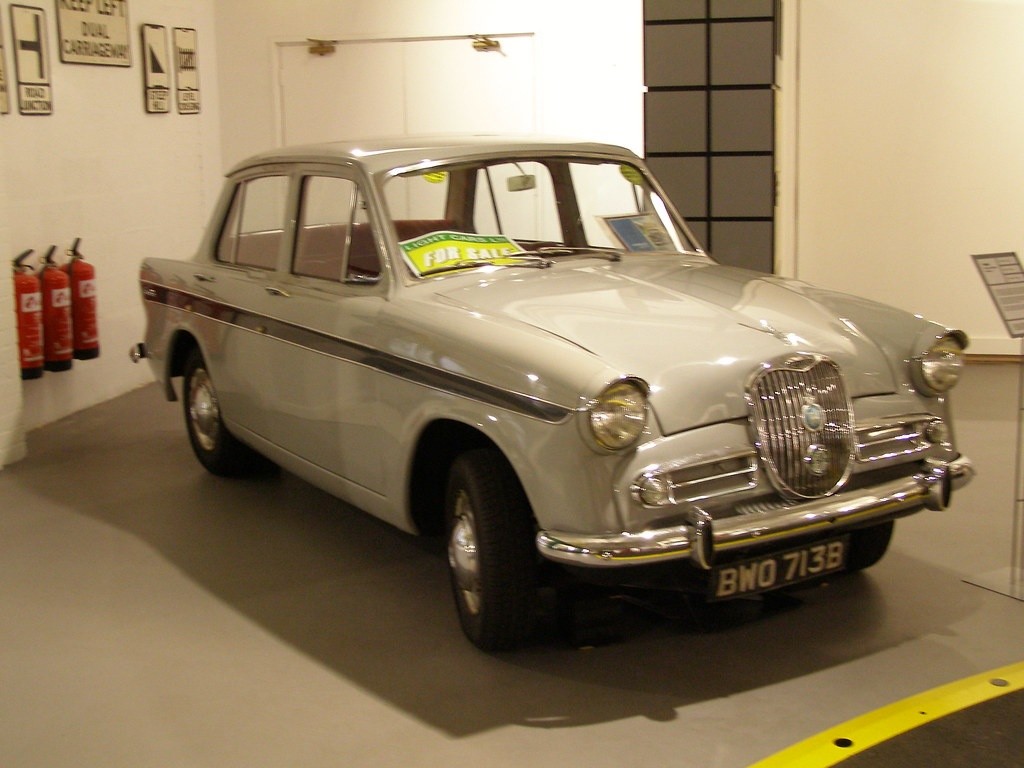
<box><xmin>129</xmin><ymin>145</ymin><xmax>983</xmax><ymax>653</ymax></box>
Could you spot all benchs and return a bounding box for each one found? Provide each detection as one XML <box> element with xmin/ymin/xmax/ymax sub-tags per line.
<box><xmin>229</xmin><ymin>220</ymin><xmax>466</xmax><ymax>278</ymax></box>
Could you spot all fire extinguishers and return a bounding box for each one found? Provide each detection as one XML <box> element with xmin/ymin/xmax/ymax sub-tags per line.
<box><xmin>12</xmin><ymin>249</ymin><xmax>43</xmax><ymax>380</ymax></box>
<box><xmin>37</xmin><ymin>246</ymin><xmax>72</xmax><ymax>373</ymax></box>
<box><xmin>57</xmin><ymin>237</ymin><xmax>100</xmax><ymax>361</ymax></box>
